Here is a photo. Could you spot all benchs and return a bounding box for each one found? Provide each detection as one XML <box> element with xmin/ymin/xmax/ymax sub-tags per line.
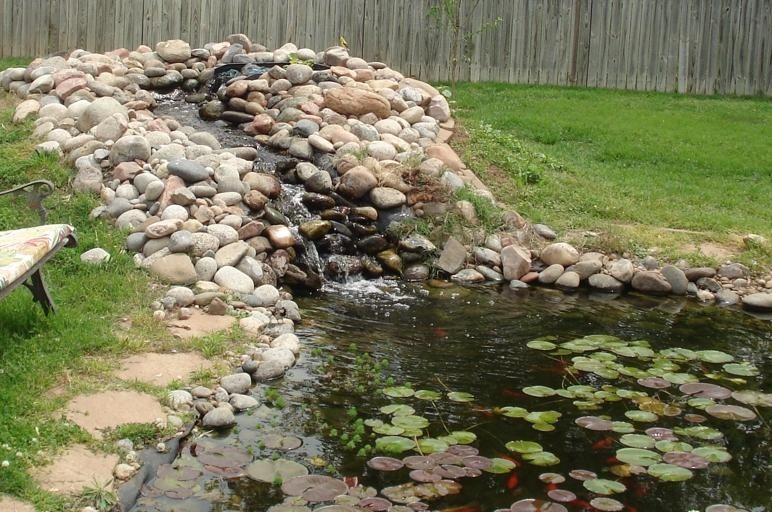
<box><xmin>1</xmin><ymin>179</ymin><xmax>79</xmax><ymax>317</ymax></box>
